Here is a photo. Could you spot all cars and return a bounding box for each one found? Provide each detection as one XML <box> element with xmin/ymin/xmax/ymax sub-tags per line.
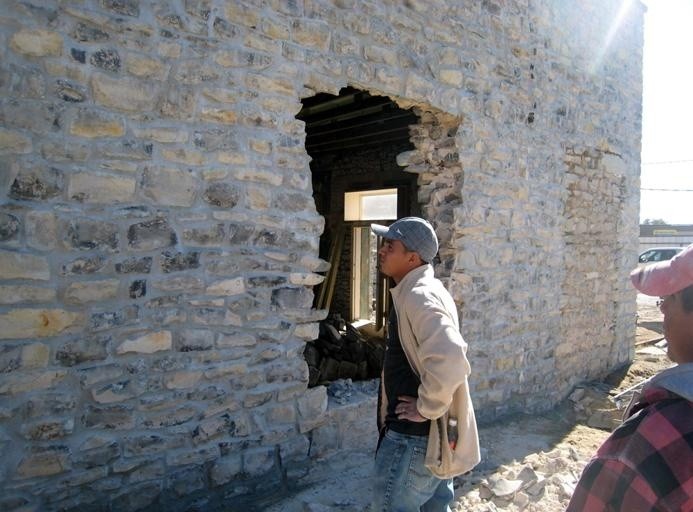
<box><xmin>638</xmin><ymin>248</ymin><xmax>685</xmax><ymax>263</ymax></box>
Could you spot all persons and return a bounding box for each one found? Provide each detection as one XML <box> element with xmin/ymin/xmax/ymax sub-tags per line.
<box><xmin>371</xmin><ymin>217</ymin><xmax>482</xmax><ymax>512</ymax></box>
<box><xmin>565</xmin><ymin>245</ymin><xmax>693</xmax><ymax>512</ymax></box>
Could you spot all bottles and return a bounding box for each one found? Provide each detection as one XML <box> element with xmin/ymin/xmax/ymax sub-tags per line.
<box><xmin>446</xmin><ymin>418</ymin><xmax>458</xmax><ymax>450</ymax></box>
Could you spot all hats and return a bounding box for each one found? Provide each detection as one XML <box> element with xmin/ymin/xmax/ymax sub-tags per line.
<box><xmin>370</xmin><ymin>217</ymin><xmax>438</xmax><ymax>263</ymax></box>
<box><xmin>630</xmin><ymin>243</ymin><xmax>693</xmax><ymax>296</ymax></box>
